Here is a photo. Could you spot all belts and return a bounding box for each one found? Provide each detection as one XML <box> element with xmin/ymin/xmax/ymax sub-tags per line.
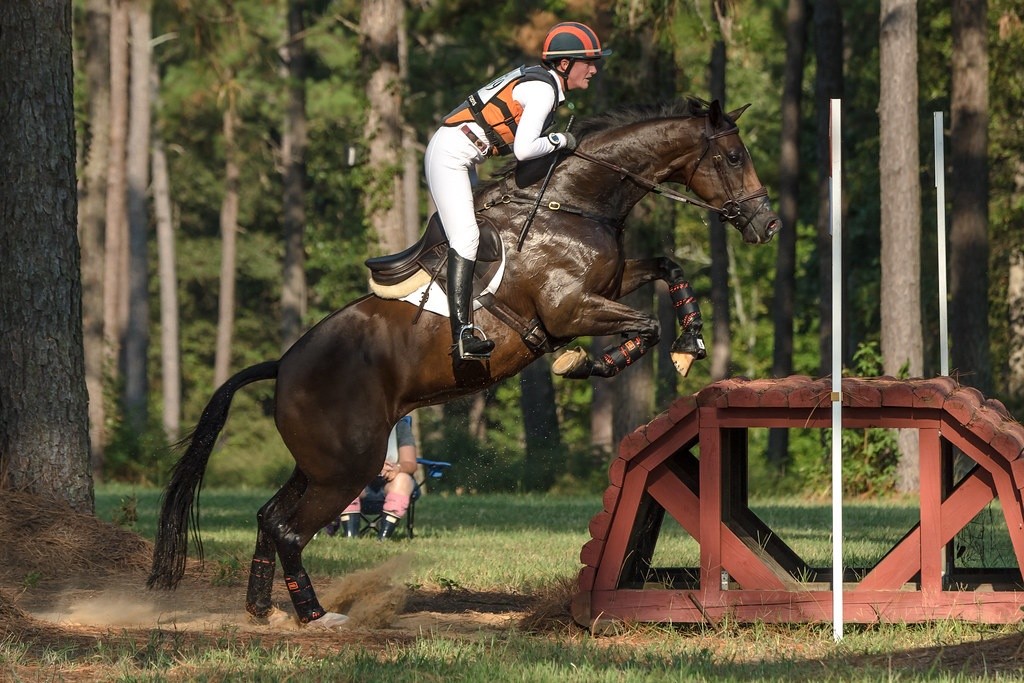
<box><xmin>460</xmin><ymin>125</ymin><xmax>494</xmax><ymax>159</ymax></box>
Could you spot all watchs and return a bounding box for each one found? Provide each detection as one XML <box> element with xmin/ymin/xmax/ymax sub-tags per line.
<box><xmin>396</xmin><ymin>463</ymin><xmax>402</xmax><ymax>472</ymax></box>
<box><xmin>547</xmin><ymin>132</ymin><xmax>561</xmax><ymax>151</ymax></box>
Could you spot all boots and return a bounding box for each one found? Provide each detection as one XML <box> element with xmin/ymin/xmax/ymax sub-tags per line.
<box><xmin>377</xmin><ymin>510</ymin><xmax>401</xmax><ymax>541</ymax></box>
<box><xmin>448</xmin><ymin>248</ymin><xmax>495</xmax><ymax>357</ymax></box>
<box><xmin>341</xmin><ymin>513</ymin><xmax>363</xmax><ymax>539</ymax></box>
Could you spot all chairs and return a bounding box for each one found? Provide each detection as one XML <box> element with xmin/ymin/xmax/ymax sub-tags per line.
<box><xmin>360</xmin><ymin>416</ymin><xmax>451</xmax><ymax>541</ymax></box>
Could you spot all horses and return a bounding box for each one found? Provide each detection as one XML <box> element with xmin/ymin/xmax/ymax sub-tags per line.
<box><xmin>143</xmin><ymin>95</ymin><xmax>784</xmax><ymax>631</ymax></box>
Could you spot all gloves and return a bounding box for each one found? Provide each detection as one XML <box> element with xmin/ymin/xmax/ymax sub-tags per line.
<box><xmin>563</xmin><ymin>131</ymin><xmax>577</xmax><ymax>150</ymax></box>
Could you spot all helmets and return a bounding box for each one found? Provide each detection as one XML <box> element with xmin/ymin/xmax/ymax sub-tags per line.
<box><xmin>542</xmin><ymin>22</ymin><xmax>612</xmax><ymax>61</ymax></box>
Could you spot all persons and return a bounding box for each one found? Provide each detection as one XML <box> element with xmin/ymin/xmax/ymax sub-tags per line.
<box><xmin>339</xmin><ymin>420</ymin><xmax>417</xmax><ymax>538</ymax></box>
<box><xmin>424</xmin><ymin>22</ymin><xmax>612</xmax><ymax>368</ymax></box>
